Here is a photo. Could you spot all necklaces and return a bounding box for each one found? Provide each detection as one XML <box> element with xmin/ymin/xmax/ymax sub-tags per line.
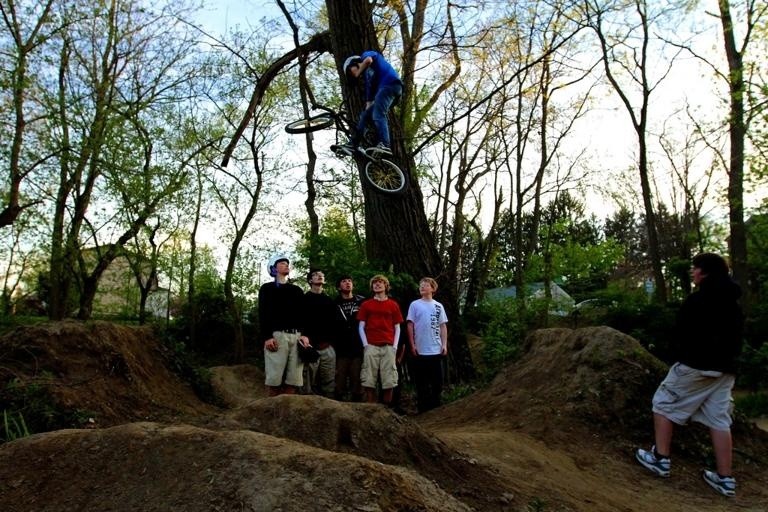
<box><xmin>374</xmin><ymin>294</ymin><xmax>387</xmax><ymax>299</ymax></box>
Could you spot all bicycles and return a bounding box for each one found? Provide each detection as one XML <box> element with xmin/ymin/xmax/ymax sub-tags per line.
<box><xmin>283</xmin><ymin>71</ymin><xmax>405</xmax><ymax>198</ymax></box>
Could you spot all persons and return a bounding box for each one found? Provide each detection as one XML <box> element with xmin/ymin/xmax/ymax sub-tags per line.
<box><xmin>355</xmin><ymin>274</ymin><xmax>405</xmax><ymax>408</ymax></box>
<box><xmin>259</xmin><ymin>256</ymin><xmax>311</xmax><ymax>396</ymax></box>
<box><xmin>330</xmin><ymin>275</ymin><xmax>369</xmax><ymax>402</ymax></box>
<box><xmin>302</xmin><ymin>269</ymin><xmax>340</xmax><ymax>398</ymax></box>
<box><xmin>636</xmin><ymin>253</ymin><xmax>745</xmax><ymax>497</ymax></box>
<box><xmin>406</xmin><ymin>277</ymin><xmax>449</xmax><ymax>413</ymax></box>
<box><xmin>330</xmin><ymin>51</ymin><xmax>404</xmax><ymax>159</ymax></box>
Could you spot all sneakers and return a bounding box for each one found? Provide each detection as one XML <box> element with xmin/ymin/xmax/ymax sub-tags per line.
<box><xmin>366</xmin><ymin>143</ymin><xmax>393</xmax><ymax>158</ymax></box>
<box><xmin>703</xmin><ymin>471</ymin><xmax>736</xmax><ymax>497</ymax></box>
<box><xmin>635</xmin><ymin>444</ymin><xmax>670</xmax><ymax>477</ymax></box>
<box><xmin>330</xmin><ymin>142</ymin><xmax>356</xmax><ymax>156</ymax></box>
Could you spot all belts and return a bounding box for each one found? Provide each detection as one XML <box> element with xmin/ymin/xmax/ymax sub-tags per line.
<box><xmin>277</xmin><ymin>329</ymin><xmax>301</xmax><ymax>334</ymax></box>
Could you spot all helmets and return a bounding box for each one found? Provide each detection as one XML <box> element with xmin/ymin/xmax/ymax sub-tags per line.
<box><xmin>344</xmin><ymin>56</ymin><xmax>361</xmax><ymax>76</ymax></box>
<box><xmin>267</xmin><ymin>255</ymin><xmax>289</xmax><ymax>277</ymax></box>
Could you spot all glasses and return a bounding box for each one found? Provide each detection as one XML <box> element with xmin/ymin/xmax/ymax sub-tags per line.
<box><xmin>312</xmin><ymin>272</ymin><xmax>325</xmax><ymax>276</ymax></box>
